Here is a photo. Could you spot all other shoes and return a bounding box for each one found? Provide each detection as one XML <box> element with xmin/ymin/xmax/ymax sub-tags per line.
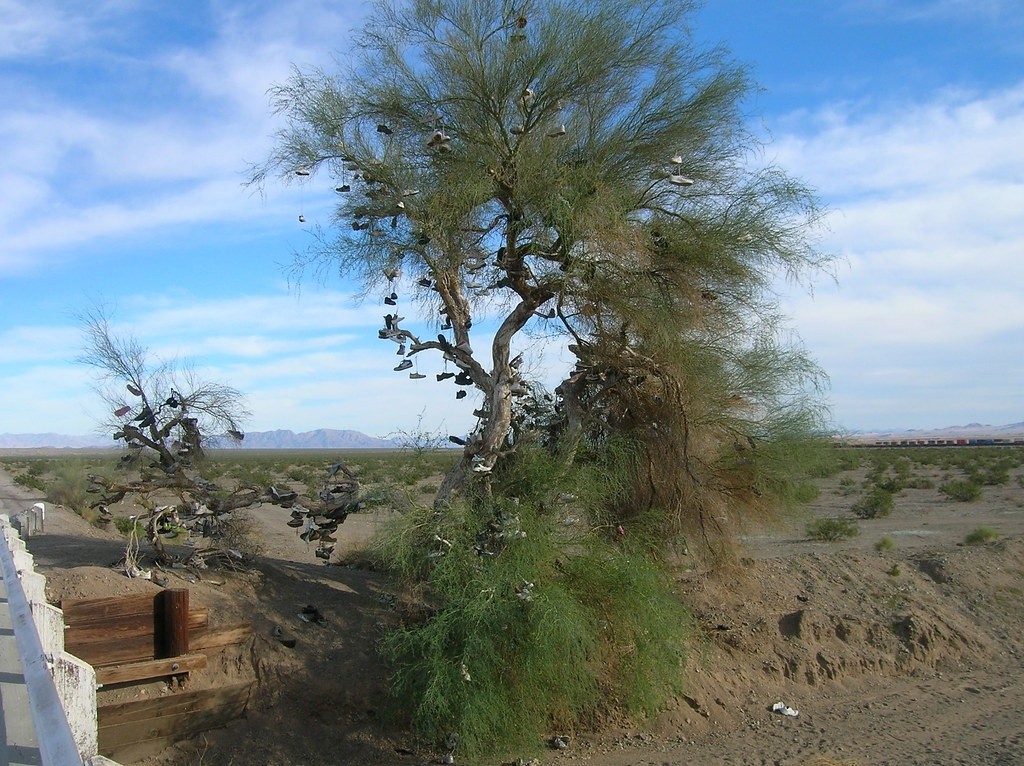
<box><xmin>295</xmin><ymin>13</ymin><xmax>796</xmax><ymax>606</ymax></box>
<box><xmin>273</xmin><ymin>624</ymin><xmax>296</xmax><ymax>648</ymax></box>
<box><xmin>515</xmin><ymin>757</ymin><xmax>542</xmax><ymax>766</ymax></box>
<box><xmin>458</xmin><ymin>664</ymin><xmax>472</xmax><ymax>683</ymax></box>
<box><xmin>445</xmin><ymin>729</ymin><xmax>460</xmax><ymax>748</ymax></box>
<box><xmin>780</xmin><ymin>705</ymin><xmax>799</xmax><ymax>716</ymax></box>
<box><xmin>154</xmin><ymin>574</ymin><xmax>169</xmax><ymax>588</ymax></box>
<box><xmin>432</xmin><ymin>754</ymin><xmax>454</xmax><ymax>765</ymax></box>
<box><xmin>773</xmin><ymin>701</ymin><xmax>785</xmax><ymax>712</ymax></box>
<box><xmin>131</xmin><ymin>570</ymin><xmax>151</xmax><ymax>580</ymax></box>
<box><xmin>267</xmin><ymin>463</ymin><xmax>367</xmax><ymax>559</ymax></box>
<box><xmin>554</xmin><ymin>734</ymin><xmax>571</xmax><ymax>749</ymax></box>
<box><xmin>86</xmin><ymin>384</ymin><xmax>262</xmax><ymax>569</ymax></box>
<box><xmin>302</xmin><ymin>605</ymin><xmax>328</xmax><ymax>628</ymax></box>
